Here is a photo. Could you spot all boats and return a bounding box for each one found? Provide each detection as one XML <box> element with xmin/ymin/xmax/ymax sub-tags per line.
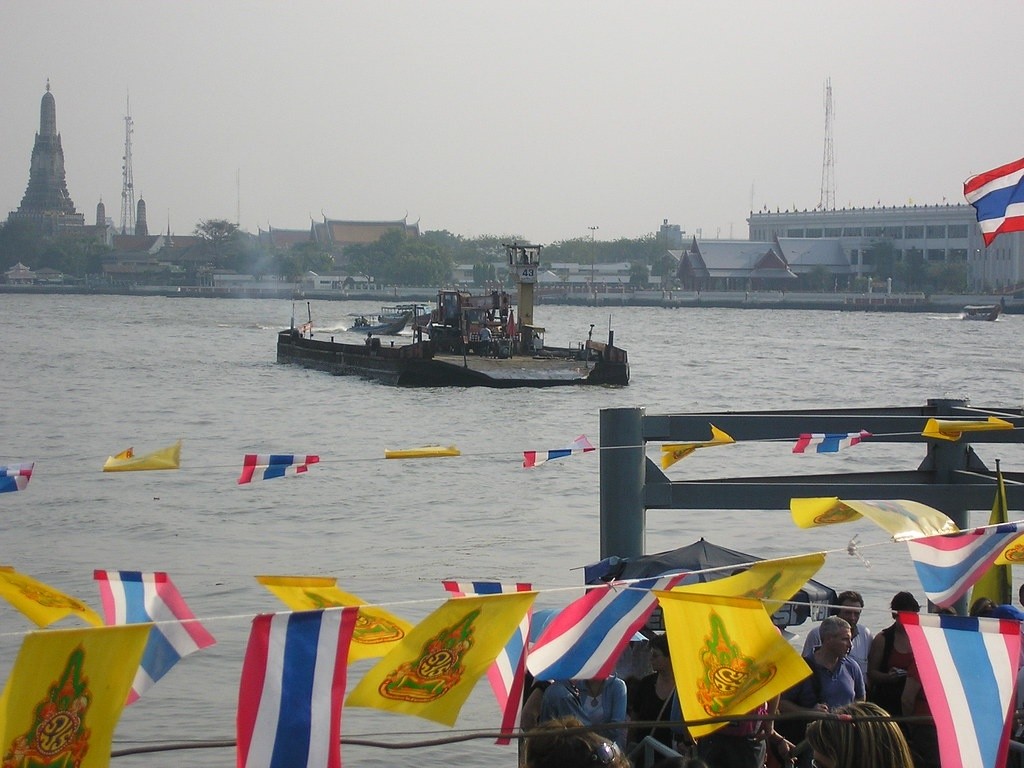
<box><xmin>962</xmin><ymin>304</ymin><xmax>1000</xmax><ymax>321</ymax></box>
<box><xmin>382</xmin><ymin>304</ymin><xmax>432</xmax><ymax>327</ymax></box>
<box><xmin>347</xmin><ymin>312</ymin><xmax>412</xmax><ymax>334</ymax></box>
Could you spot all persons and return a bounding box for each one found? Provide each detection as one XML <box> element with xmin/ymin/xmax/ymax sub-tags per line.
<box><xmin>479</xmin><ymin>324</ymin><xmax>492</xmax><ymax>357</ymax></box>
<box><xmin>524</xmin><ymin>584</ymin><xmax>1024</xmax><ymax>768</ymax></box>
<box><xmin>520</xmin><ymin>250</ymin><xmax>528</xmax><ymax>263</ymax></box>
<box><xmin>364</xmin><ymin>332</ymin><xmax>373</xmax><ymax>347</ymax></box>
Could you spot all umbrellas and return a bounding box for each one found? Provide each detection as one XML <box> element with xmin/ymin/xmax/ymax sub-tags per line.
<box><xmin>584</xmin><ymin>537</ymin><xmax>837</xmax><ymax>625</ymax></box>
<box><xmin>506</xmin><ymin>311</ymin><xmax>515</xmax><ymax>338</ymax></box>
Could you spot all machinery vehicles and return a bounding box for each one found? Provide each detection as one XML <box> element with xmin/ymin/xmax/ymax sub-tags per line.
<box><xmin>410</xmin><ymin>288</ymin><xmax>522</xmax><ymax>358</ymax></box>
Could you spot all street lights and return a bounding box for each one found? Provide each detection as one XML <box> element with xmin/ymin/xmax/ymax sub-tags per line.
<box><xmin>740</xmin><ymin>251</ymin><xmax>761</xmax><ymax>291</ymax></box>
<box><xmin>902</xmin><ymin>249</ymin><xmax>922</xmax><ymax>290</ymax></box>
<box><xmin>791</xmin><ymin>251</ymin><xmax>809</xmax><ymax>290</ymax></box>
<box><xmin>588</xmin><ymin>226</ymin><xmax>600</xmax><ymax>284</ymax></box>
<box><xmin>977</xmin><ymin>250</ymin><xmax>994</xmax><ymax>290</ymax></box>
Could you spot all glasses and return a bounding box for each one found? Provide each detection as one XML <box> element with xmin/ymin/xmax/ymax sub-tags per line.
<box><xmin>589</xmin><ymin>741</ymin><xmax>621</xmax><ymax>768</ymax></box>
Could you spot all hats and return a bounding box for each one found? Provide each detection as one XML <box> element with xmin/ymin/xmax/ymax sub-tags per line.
<box><xmin>993</xmin><ymin>604</ymin><xmax>1024</xmax><ymax>621</ymax></box>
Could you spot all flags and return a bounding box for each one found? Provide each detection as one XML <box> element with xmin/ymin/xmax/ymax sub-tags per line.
<box><xmin>897</xmin><ymin>611</ymin><xmax>1021</xmax><ymax>768</ymax></box>
<box><xmin>0</xmin><ymin>549</ymin><xmax>828</xmax><ymax>768</ymax></box>
<box><xmin>963</xmin><ymin>157</ymin><xmax>1024</xmax><ymax>248</ymax></box>
<box><xmin>968</xmin><ymin>474</ymin><xmax>1012</xmax><ymax>613</ymax></box>
<box><xmin>102</xmin><ymin>440</ymin><xmax>181</xmax><ymax>472</ymax></box>
<box><xmin>236</xmin><ymin>453</ymin><xmax>319</xmax><ymax>484</ymax></box>
<box><xmin>0</xmin><ymin>460</ymin><xmax>34</xmax><ymax>494</ymax></box>
<box><xmin>789</xmin><ymin>497</ymin><xmax>961</xmax><ymax>543</ymax></box>
<box><xmin>921</xmin><ymin>415</ymin><xmax>1014</xmax><ymax>442</ymax></box>
<box><xmin>908</xmin><ymin>522</ymin><xmax>1024</xmax><ymax>608</ymax></box>
<box><xmin>523</xmin><ymin>434</ymin><xmax>595</xmax><ymax>468</ymax></box>
<box><xmin>793</xmin><ymin>429</ymin><xmax>873</xmax><ymax>453</ymax></box>
<box><xmin>994</xmin><ymin>534</ymin><xmax>1024</xmax><ymax>565</ymax></box>
<box><xmin>385</xmin><ymin>444</ymin><xmax>460</xmax><ymax>459</ymax></box>
<box><xmin>660</xmin><ymin>422</ymin><xmax>736</xmax><ymax>471</ymax></box>
